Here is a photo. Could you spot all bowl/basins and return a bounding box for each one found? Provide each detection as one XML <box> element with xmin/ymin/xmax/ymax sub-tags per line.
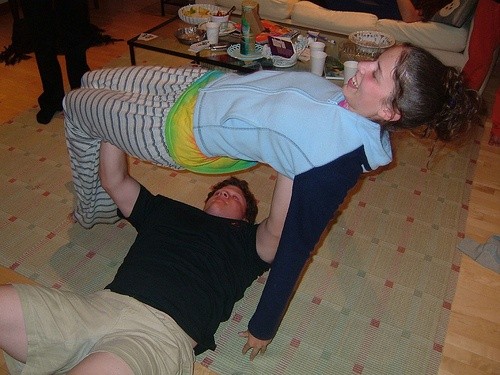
<box><xmin>175</xmin><ymin>28</ymin><xmax>206</xmax><ymax>44</ymax></box>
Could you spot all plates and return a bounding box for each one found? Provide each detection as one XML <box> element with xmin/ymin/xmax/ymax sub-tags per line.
<box><xmin>198</xmin><ymin>20</ymin><xmax>237</xmax><ymax>36</ymax></box>
<box><xmin>349</xmin><ymin>31</ymin><xmax>396</xmax><ymax>49</ymax></box>
<box><xmin>178</xmin><ymin>4</ymin><xmax>222</xmax><ymax>25</ymax></box>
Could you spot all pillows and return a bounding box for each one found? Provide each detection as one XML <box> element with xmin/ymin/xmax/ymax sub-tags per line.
<box><xmin>431</xmin><ymin>0</ymin><xmax>478</xmax><ymax>29</ymax></box>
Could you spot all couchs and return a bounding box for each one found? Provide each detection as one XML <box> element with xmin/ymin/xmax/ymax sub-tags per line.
<box><xmin>196</xmin><ymin>0</ymin><xmax>499</xmax><ymax>95</ymax></box>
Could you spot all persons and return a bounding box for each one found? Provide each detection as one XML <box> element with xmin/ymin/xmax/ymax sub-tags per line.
<box><xmin>308</xmin><ymin>0</ymin><xmax>454</xmax><ymax>23</ymax></box>
<box><xmin>1</xmin><ymin>0</ymin><xmax>123</xmax><ymax>124</ymax></box>
<box><xmin>61</xmin><ymin>42</ymin><xmax>482</xmax><ymax>360</ymax></box>
<box><xmin>0</xmin><ymin>141</ymin><xmax>295</xmax><ymax>375</ymax></box>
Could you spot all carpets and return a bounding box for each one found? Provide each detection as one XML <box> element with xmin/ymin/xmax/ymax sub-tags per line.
<box><xmin>0</xmin><ymin>48</ymin><xmax>500</xmax><ymax>375</ymax></box>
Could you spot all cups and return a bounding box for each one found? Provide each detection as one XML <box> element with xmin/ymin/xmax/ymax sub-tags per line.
<box><xmin>206</xmin><ymin>22</ymin><xmax>219</xmax><ymax>44</ymax></box>
<box><xmin>210</xmin><ymin>11</ymin><xmax>229</xmax><ymax>31</ymax></box>
<box><xmin>343</xmin><ymin>60</ymin><xmax>359</xmax><ymax>85</ymax></box>
<box><xmin>310</xmin><ymin>41</ymin><xmax>326</xmax><ymax>52</ymax></box>
<box><xmin>310</xmin><ymin>51</ymin><xmax>327</xmax><ymax>76</ymax></box>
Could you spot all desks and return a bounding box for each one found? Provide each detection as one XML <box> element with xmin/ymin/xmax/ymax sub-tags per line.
<box><xmin>127</xmin><ymin>16</ymin><xmax>377</xmax><ymax>88</ymax></box>
